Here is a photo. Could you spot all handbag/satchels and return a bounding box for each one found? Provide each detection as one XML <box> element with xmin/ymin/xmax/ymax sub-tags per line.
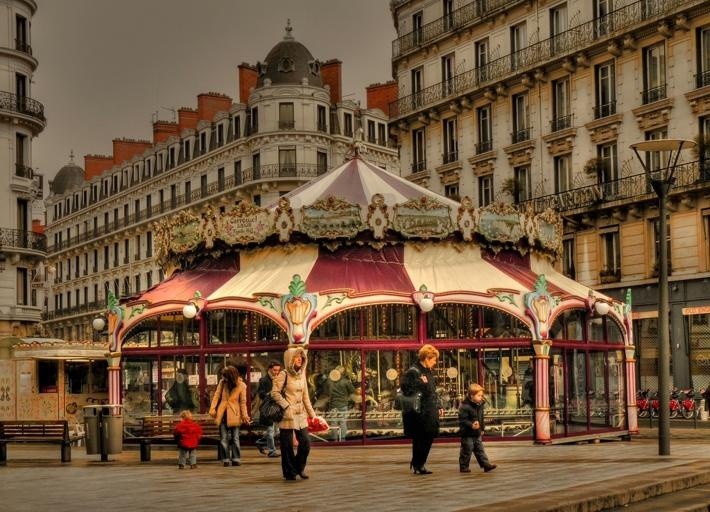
<box><xmin>259</xmin><ymin>397</ymin><xmax>283</xmax><ymax>422</ymax></box>
<box><xmin>393</xmin><ymin>389</ymin><xmax>424</xmax><ymax>414</ymax></box>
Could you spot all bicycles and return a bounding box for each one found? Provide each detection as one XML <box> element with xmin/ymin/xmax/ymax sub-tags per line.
<box><xmin>559</xmin><ymin>386</ymin><xmax>699</xmax><ymax>420</ymax></box>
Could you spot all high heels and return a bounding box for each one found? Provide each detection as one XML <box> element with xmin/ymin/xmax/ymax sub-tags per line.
<box><xmin>410</xmin><ymin>462</ymin><xmax>433</xmax><ymax>474</ymax></box>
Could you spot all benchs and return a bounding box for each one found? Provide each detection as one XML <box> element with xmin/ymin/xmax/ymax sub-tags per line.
<box><xmin>0</xmin><ymin>420</ymin><xmax>71</xmax><ymax>465</ymax></box>
<box><xmin>123</xmin><ymin>414</ymin><xmax>231</xmax><ymax>462</ymax></box>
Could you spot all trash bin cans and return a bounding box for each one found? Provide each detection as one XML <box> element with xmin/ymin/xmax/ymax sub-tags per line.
<box><xmin>83</xmin><ymin>404</ymin><xmax>124</xmax><ymax>455</ymax></box>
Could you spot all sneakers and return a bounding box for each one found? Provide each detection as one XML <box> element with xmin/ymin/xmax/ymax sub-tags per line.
<box><xmin>253</xmin><ymin>441</ymin><xmax>281</xmax><ymax>457</ymax></box>
<box><xmin>484</xmin><ymin>464</ymin><xmax>496</xmax><ymax>472</ymax></box>
<box><xmin>299</xmin><ymin>471</ymin><xmax>309</xmax><ymax>478</ymax></box>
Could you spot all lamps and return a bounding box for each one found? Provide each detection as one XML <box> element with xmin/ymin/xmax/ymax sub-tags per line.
<box><xmin>183</xmin><ymin>302</ymin><xmax>199</xmax><ymax>318</ymax></box>
<box><xmin>93</xmin><ymin>315</ymin><xmax>108</xmax><ymax>336</ymax></box>
<box><xmin>592</xmin><ymin>300</ymin><xmax>609</xmax><ymax>315</ymax></box>
<box><xmin>420</xmin><ymin>295</ymin><xmax>434</xmax><ymax>312</ymax></box>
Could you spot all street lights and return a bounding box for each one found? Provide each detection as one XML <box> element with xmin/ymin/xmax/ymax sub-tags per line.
<box><xmin>629</xmin><ymin>139</ymin><xmax>697</xmax><ymax>456</ymax></box>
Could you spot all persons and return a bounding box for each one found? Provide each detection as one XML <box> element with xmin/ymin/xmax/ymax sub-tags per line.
<box><xmin>209</xmin><ymin>365</ymin><xmax>251</xmax><ymax>468</ymax></box>
<box><xmin>327</xmin><ymin>366</ymin><xmax>356</xmax><ymax>443</ymax></box>
<box><xmin>256</xmin><ymin>361</ymin><xmax>281</xmax><ymax>458</ymax></box>
<box><xmin>172</xmin><ymin>411</ymin><xmax>205</xmax><ymax>468</ymax></box>
<box><xmin>394</xmin><ymin>344</ymin><xmax>445</xmax><ymax>476</ymax></box>
<box><xmin>270</xmin><ymin>346</ymin><xmax>317</xmax><ymax>480</ymax></box>
<box><xmin>457</xmin><ymin>383</ymin><xmax>497</xmax><ymax>472</ymax></box>
